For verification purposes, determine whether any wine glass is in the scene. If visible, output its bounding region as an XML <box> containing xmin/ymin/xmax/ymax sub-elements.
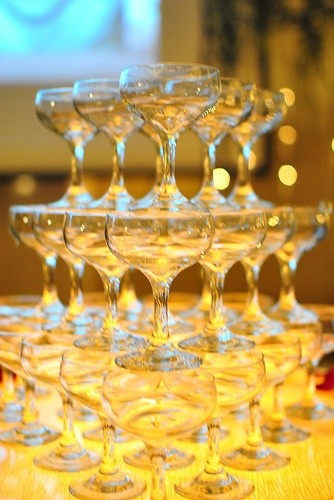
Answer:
<box><xmin>70</xmin><ymin>78</ymin><xmax>143</xmax><ymax>211</ymax></box>
<box><xmin>190</xmin><ymin>76</ymin><xmax>257</xmax><ymax>210</ymax></box>
<box><xmin>0</xmin><ymin>201</ymin><xmax>334</xmax><ymax>500</ymax></box>
<box><xmin>227</xmin><ymin>86</ymin><xmax>287</xmax><ymax>210</ymax></box>
<box><xmin>118</xmin><ymin>62</ymin><xmax>220</xmax><ymax>212</ymax></box>
<box><xmin>36</xmin><ymin>87</ymin><xmax>102</xmax><ymax>210</ymax></box>
